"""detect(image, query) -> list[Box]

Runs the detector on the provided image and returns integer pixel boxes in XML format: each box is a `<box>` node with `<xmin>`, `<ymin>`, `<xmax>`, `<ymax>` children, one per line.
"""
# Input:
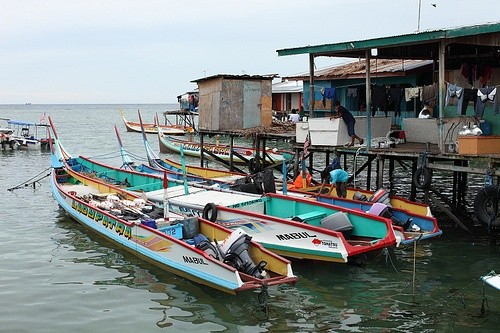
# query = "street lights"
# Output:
<box><xmin>416</xmin><ymin>0</ymin><xmax>437</xmax><ymax>32</ymax></box>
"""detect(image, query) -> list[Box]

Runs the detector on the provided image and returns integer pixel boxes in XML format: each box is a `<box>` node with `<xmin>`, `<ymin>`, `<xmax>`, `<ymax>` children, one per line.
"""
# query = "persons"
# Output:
<box><xmin>289</xmin><ymin>106</ymin><xmax>305</xmax><ymax>123</ymax></box>
<box><xmin>0</xmin><ymin>130</ymin><xmax>16</xmax><ymax>155</ymax></box>
<box><xmin>315</xmin><ymin>169</ymin><xmax>348</xmax><ymax>198</ymax></box>
<box><xmin>330</xmin><ymin>101</ymin><xmax>363</xmax><ymax>146</ymax></box>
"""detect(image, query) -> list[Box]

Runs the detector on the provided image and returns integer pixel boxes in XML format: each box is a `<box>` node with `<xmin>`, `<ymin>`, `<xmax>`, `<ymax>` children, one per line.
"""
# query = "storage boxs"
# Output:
<box><xmin>457</xmin><ymin>135</ymin><xmax>500</xmax><ymax>155</ymax></box>
<box><xmin>157</xmin><ymin>223</ymin><xmax>184</xmax><ymax>239</ymax></box>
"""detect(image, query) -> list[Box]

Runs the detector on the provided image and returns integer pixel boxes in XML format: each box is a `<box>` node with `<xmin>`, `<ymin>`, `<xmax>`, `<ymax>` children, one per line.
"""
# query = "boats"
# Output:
<box><xmin>0</xmin><ymin>117</ymin><xmax>55</xmax><ymax>151</ymax></box>
<box><xmin>118</xmin><ymin>111</ymin><xmax>194</xmax><ymax>135</ymax></box>
<box><xmin>155</xmin><ymin>111</ymin><xmax>297</xmax><ymax>166</ymax></box>
<box><xmin>48</xmin><ymin>108</ymin><xmax>444</xmax><ymax>296</ymax></box>
<box><xmin>480</xmin><ymin>270</ymin><xmax>500</xmax><ymax>291</ymax></box>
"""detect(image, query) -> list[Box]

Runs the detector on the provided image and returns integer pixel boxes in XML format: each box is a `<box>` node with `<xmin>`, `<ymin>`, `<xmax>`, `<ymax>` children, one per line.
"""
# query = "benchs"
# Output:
<box><xmin>288</xmin><ymin>211</ymin><xmax>326</xmax><ymax>226</ymax></box>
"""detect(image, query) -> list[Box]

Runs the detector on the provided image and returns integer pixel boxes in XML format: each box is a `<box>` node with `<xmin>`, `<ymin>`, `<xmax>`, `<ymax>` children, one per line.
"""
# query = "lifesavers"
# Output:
<box><xmin>202</xmin><ymin>203</ymin><xmax>217</xmax><ymax>223</ymax></box>
<box><xmin>413</xmin><ymin>168</ymin><xmax>431</xmax><ymax>188</ymax></box>
<box><xmin>248</xmin><ymin>158</ymin><xmax>259</xmax><ymax>175</ymax></box>
<box><xmin>474</xmin><ymin>185</ymin><xmax>500</xmax><ymax>230</ymax></box>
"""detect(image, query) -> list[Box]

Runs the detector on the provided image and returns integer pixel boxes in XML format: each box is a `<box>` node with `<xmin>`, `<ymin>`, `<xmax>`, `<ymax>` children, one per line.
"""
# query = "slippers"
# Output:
<box><xmin>360</xmin><ymin>139</ymin><xmax>363</xmax><ymax>145</ymax></box>
